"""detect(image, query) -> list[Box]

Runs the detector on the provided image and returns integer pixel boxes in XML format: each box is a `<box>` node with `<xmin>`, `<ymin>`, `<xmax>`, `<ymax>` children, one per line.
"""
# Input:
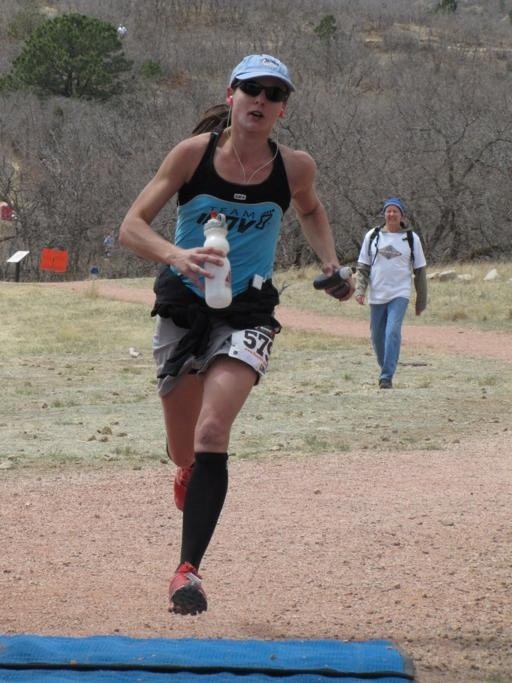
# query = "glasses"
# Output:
<box><xmin>234</xmin><ymin>80</ymin><xmax>289</xmax><ymax>102</ymax></box>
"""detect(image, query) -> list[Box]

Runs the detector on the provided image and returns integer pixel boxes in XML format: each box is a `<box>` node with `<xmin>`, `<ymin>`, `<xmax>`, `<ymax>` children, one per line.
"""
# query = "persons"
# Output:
<box><xmin>354</xmin><ymin>198</ymin><xmax>428</xmax><ymax>388</ymax></box>
<box><xmin>118</xmin><ymin>52</ymin><xmax>358</xmax><ymax>616</ymax></box>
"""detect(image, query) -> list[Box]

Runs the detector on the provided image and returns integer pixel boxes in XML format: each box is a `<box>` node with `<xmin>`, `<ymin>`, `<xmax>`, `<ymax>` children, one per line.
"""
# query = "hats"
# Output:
<box><xmin>229</xmin><ymin>54</ymin><xmax>297</xmax><ymax>93</ymax></box>
<box><xmin>382</xmin><ymin>198</ymin><xmax>405</xmax><ymax>217</ymax></box>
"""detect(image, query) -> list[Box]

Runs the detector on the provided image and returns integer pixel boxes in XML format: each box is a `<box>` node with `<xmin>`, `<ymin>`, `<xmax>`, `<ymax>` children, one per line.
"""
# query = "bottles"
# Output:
<box><xmin>199</xmin><ymin>208</ymin><xmax>233</xmax><ymax>314</ymax></box>
<box><xmin>311</xmin><ymin>266</ymin><xmax>357</xmax><ymax>290</ymax></box>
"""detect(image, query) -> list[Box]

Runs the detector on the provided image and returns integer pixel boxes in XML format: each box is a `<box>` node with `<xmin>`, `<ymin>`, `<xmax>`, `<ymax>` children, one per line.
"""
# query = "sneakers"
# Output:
<box><xmin>168</xmin><ymin>561</ymin><xmax>207</xmax><ymax>616</ymax></box>
<box><xmin>174</xmin><ymin>461</ymin><xmax>195</xmax><ymax>512</ymax></box>
<box><xmin>379</xmin><ymin>378</ymin><xmax>392</xmax><ymax>388</ymax></box>
